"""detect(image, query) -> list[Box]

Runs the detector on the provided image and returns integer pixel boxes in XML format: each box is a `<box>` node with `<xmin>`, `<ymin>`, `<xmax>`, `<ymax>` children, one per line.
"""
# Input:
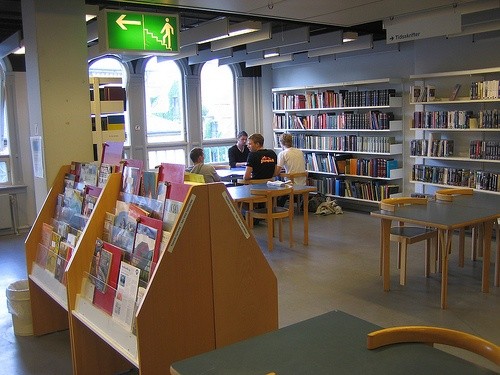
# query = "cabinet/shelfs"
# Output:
<box><xmin>410</xmin><ymin>67</ymin><xmax>500</xmax><ymax>194</ymax></box>
<box><xmin>272</xmin><ymin>78</ymin><xmax>405</xmax><ymax>204</ymax></box>
<box><xmin>88</xmin><ymin>76</ymin><xmax>126</xmax><ymax>162</ymax></box>
<box><xmin>24</xmin><ymin>164</ymin><xmax>277</xmax><ymax>375</ymax></box>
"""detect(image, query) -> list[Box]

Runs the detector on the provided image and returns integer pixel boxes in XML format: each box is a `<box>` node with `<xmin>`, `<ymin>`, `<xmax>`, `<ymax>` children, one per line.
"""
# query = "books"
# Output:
<box><xmin>409</xmin><ymin>80</ymin><xmax>500</xmax><ymax>191</ymax></box>
<box><xmin>35</xmin><ymin>86</ymin><xmax>192</xmax><ymax>334</ymax></box>
<box><xmin>271</xmin><ymin>88</ymin><xmax>399</xmax><ymax>201</ymax></box>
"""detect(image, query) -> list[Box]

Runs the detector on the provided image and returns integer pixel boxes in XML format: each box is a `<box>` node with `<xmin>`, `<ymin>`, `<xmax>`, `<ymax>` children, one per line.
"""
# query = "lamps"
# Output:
<box><xmin>343</xmin><ymin>32</ymin><xmax>358</xmax><ymax>43</ymax></box>
<box><xmin>196</xmin><ymin>20</ymin><xmax>262</xmax><ymax>45</ymax></box>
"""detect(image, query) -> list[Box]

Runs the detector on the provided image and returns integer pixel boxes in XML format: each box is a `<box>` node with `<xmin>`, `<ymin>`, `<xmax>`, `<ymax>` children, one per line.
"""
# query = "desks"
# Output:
<box><xmin>225</xmin><ymin>182</ymin><xmax>318</xmax><ymax>246</ymax></box>
<box><xmin>169</xmin><ymin>311</ymin><xmax>500</xmax><ymax>375</ymax></box>
<box><xmin>0</xmin><ymin>184</ymin><xmax>27</xmax><ymax>236</ymax></box>
<box><xmin>214</xmin><ymin>168</ymin><xmax>245</xmax><ymax>176</ymax></box>
<box><xmin>370</xmin><ymin>192</ymin><xmax>500</xmax><ymax>309</ymax></box>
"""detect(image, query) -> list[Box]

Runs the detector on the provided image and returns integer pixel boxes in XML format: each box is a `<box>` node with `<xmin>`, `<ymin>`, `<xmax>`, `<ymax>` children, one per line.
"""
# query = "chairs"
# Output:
<box><xmin>236</xmin><ymin>171</ymin><xmax>309</xmax><ymax>252</ymax></box>
<box><xmin>367</xmin><ymin>326</ymin><xmax>500</xmax><ymax>366</ymax></box>
<box><xmin>380</xmin><ymin>188</ymin><xmax>484</xmax><ymax>286</ymax></box>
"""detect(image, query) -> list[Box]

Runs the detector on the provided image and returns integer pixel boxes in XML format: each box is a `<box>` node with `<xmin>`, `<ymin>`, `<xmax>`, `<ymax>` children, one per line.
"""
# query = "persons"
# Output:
<box><xmin>228</xmin><ymin>130</ymin><xmax>250</xmax><ymax>182</ymax></box>
<box><xmin>190</xmin><ymin>147</ymin><xmax>220</xmax><ymax>183</ymax></box>
<box><xmin>242</xmin><ymin>133</ymin><xmax>277</xmax><ymax>224</ymax></box>
<box><xmin>277</xmin><ymin>133</ymin><xmax>306</xmax><ymax>206</ymax></box>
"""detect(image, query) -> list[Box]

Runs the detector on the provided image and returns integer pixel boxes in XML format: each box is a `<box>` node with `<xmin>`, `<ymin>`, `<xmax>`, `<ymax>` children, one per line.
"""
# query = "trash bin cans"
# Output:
<box><xmin>6</xmin><ymin>279</ymin><xmax>34</xmax><ymax>337</ymax></box>
